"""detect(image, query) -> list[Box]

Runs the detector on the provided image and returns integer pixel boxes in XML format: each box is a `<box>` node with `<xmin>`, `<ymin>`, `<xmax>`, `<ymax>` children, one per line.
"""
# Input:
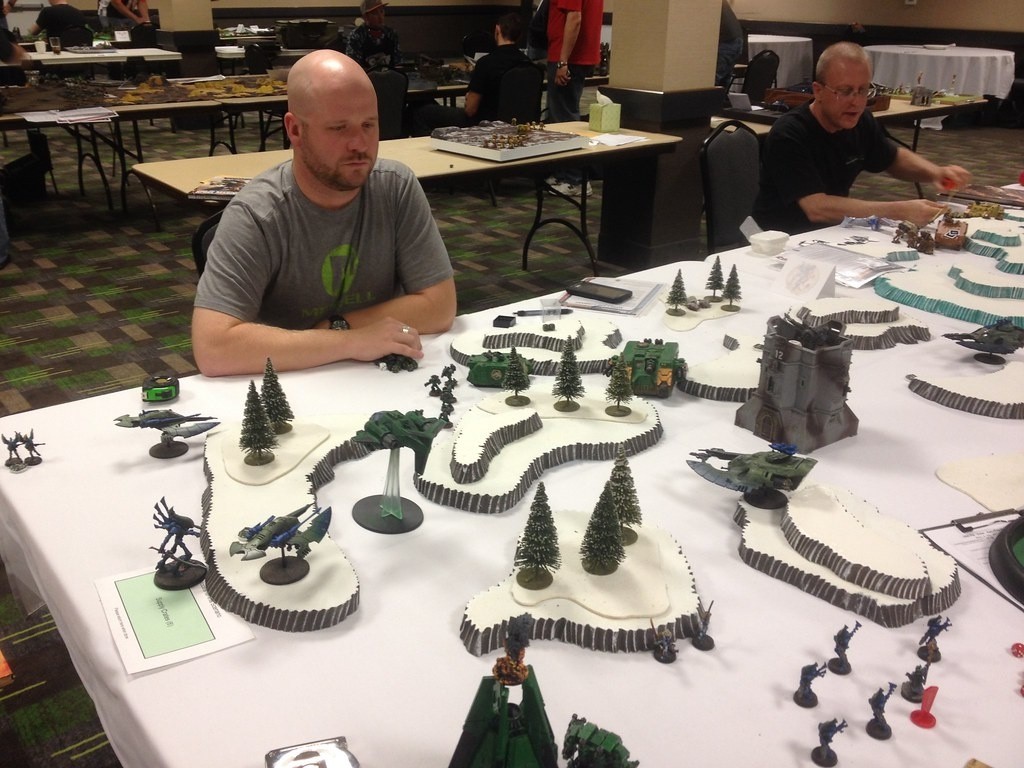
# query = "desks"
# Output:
<box><xmin>866</xmin><ymin>44</ymin><xmax>1017</xmax><ymax>132</ymax></box>
<box><xmin>132</xmin><ymin>119</ymin><xmax>686</xmax><ymax>280</ymax></box>
<box><xmin>0</xmin><ymin>85</ymin><xmax>223</xmax><ymax>224</ymax></box>
<box><xmin>710</xmin><ymin>97</ymin><xmax>989</xmax><ymax>221</ymax></box>
<box><xmin>747</xmin><ymin>32</ymin><xmax>813</xmax><ymax>90</ymax></box>
<box><xmin>215</xmin><ymin>43</ymin><xmax>318</xmax><ymax>57</ymax></box>
<box><xmin>205</xmin><ymin>72</ymin><xmax>292</xmax><ymax>156</ymax></box>
<box><xmin>217</xmin><ymin>35</ymin><xmax>276</xmax><ymax>42</ymax></box>
<box><xmin>38</xmin><ymin>46</ymin><xmax>184</xmax><ymax>80</ymax></box>
<box><xmin>0</xmin><ymin>184</ymin><xmax>1024</xmax><ymax>768</ymax></box>
<box><xmin>404</xmin><ymin>76</ymin><xmax>609</xmax><ymax>107</ymax></box>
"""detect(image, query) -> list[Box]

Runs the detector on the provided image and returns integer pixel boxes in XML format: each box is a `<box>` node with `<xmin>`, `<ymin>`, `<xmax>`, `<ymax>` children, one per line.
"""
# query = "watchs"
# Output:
<box><xmin>328</xmin><ymin>314</ymin><xmax>350</xmax><ymax>330</ymax></box>
<box><xmin>557</xmin><ymin>60</ymin><xmax>567</xmax><ymax>68</ymax></box>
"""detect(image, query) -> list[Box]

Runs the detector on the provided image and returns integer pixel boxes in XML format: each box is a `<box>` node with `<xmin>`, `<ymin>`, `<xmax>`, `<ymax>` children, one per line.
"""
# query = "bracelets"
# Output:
<box><xmin>8</xmin><ymin>1</ymin><xmax>14</xmax><ymax>8</ymax></box>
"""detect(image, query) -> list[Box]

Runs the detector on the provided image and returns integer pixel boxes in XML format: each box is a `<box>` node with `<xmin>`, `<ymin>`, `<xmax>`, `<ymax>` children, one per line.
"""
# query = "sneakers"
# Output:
<box><xmin>542</xmin><ymin>176</ymin><xmax>569</xmax><ymax>193</ymax></box>
<box><xmin>549</xmin><ymin>181</ymin><xmax>593</xmax><ymax>196</ymax></box>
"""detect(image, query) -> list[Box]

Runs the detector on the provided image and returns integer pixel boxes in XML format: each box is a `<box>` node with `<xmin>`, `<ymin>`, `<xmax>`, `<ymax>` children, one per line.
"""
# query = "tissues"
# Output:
<box><xmin>588</xmin><ymin>89</ymin><xmax>621</xmax><ymax>133</ymax></box>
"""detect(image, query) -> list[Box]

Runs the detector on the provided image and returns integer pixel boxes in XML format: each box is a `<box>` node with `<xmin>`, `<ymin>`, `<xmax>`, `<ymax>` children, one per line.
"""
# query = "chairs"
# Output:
<box><xmin>700</xmin><ymin>121</ymin><xmax>766</xmax><ymax>249</ymax></box>
<box><xmin>446</xmin><ymin>58</ymin><xmax>542</xmax><ymax>206</ymax></box>
<box><xmin>59</xmin><ymin>26</ymin><xmax>94</xmax><ymax>49</ymax></box>
<box><xmin>130</xmin><ymin>22</ymin><xmax>160</xmax><ymax>46</ymax></box>
<box><xmin>243</xmin><ymin>42</ymin><xmax>273</xmax><ymax>75</ymax></box>
<box><xmin>191</xmin><ymin>201</ymin><xmax>230</xmax><ymax>278</ymax></box>
<box><xmin>368</xmin><ymin>64</ymin><xmax>409</xmax><ymax>137</ymax></box>
<box><xmin>734</xmin><ymin>47</ymin><xmax>782</xmax><ymax>99</ymax></box>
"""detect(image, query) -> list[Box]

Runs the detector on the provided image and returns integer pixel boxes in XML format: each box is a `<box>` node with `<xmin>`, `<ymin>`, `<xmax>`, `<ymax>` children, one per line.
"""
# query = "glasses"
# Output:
<box><xmin>818</xmin><ymin>80</ymin><xmax>877</xmax><ymax>105</ymax></box>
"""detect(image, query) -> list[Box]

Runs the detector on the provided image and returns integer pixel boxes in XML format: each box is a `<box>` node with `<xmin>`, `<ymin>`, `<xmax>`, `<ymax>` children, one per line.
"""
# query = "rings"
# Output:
<box><xmin>402</xmin><ymin>325</ymin><xmax>409</xmax><ymax>334</ymax></box>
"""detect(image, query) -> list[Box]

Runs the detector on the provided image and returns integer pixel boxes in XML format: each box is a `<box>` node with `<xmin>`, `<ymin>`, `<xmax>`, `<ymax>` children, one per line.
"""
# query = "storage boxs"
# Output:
<box><xmin>274</xmin><ymin>17</ymin><xmax>340</xmax><ymax>47</ymax></box>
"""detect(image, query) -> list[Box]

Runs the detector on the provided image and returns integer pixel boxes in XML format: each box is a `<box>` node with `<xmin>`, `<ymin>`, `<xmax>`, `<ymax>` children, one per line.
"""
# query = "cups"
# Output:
<box><xmin>49</xmin><ymin>36</ymin><xmax>61</xmax><ymax>55</ymax></box>
<box><xmin>34</xmin><ymin>41</ymin><xmax>46</xmax><ymax>54</ymax></box>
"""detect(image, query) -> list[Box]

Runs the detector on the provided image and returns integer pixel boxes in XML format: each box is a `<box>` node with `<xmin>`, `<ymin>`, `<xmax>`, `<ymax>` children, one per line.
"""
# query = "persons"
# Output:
<box><xmin>97</xmin><ymin>0</ymin><xmax>150</xmax><ymax>29</ymax></box>
<box><xmin>413</xmin><ymin>12</ymin><xmax>543</xmax><ymax>137</ymax></box>
<box><xmin>192</xmin><ymin>49</ymin><xmax>456</xmax><ymax>377</ymax></box>
<box><xmin>751</xmin><ymin>41</ymin><xmax>971</xmax><ymax>228</ymax></box>
<box><xmin>31</xmin><ymin>0</ymin><xmax>86</xmax><ymax>51</ymax></box>
<box><xmin>345</xmin><ymin>0</ymin><xmax>402</xmax><ymax>73</ymax></box>
<box><xmin>538</xmin><ymin>0</ymin><xmax>604</xmax><ymax>123</ymax></box>
<box><xmin>0</xmin><ymin>0</ymin><xmax>26</xmax><ymax>64</ymax></box>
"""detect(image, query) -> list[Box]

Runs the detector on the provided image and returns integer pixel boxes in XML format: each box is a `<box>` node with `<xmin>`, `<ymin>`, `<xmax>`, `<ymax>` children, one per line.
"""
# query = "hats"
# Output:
<box><xmin>361</xmin><ymin>0</ymin><xmax>389</xmax><ymax>15</ymax></box>
